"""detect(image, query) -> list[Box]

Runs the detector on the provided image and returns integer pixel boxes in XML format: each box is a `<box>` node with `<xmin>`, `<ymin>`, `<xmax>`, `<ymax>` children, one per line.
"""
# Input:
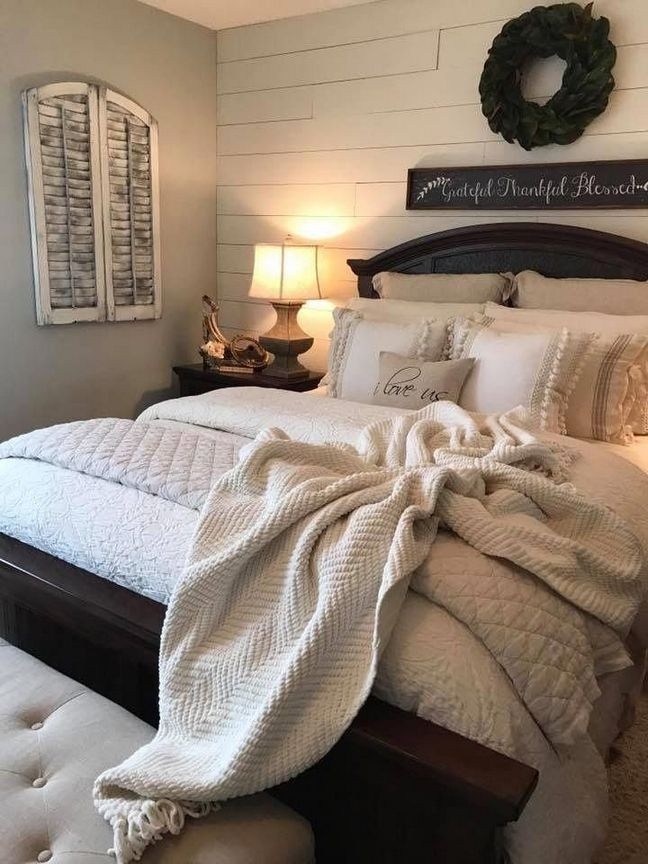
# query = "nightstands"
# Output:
<box><xmin>172</xmin><ymin>364</ymin><xmax>326</xmax><ymax>398</ymax></box>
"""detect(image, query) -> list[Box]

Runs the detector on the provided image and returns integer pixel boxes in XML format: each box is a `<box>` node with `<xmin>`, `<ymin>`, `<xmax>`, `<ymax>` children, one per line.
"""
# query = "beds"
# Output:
<box><xmin>0</xmin><ymin>222</ymin><xmax>648</xmax><ymax>864</ymax></box>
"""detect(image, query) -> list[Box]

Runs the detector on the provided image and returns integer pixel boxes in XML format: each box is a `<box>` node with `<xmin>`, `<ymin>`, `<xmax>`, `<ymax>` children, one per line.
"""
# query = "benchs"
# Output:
<box><xmin>0</xmin><ymin>637</ymin><xmax>317</xmax><ymax>864</ymax></box>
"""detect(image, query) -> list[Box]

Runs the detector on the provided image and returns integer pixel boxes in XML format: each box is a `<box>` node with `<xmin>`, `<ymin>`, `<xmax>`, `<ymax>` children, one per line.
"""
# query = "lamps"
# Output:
<box><xmin>247</xmin><ymin>234</ymin><xmax>329</xmax><ymax>378</ymax></box>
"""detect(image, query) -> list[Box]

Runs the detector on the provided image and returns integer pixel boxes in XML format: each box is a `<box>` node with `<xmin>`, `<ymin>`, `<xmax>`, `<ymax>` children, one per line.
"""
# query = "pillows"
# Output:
<box><xmin>318</xmin><ymin>270</ymin><xmax>647</xmax><ymax>445</ymax></box>
